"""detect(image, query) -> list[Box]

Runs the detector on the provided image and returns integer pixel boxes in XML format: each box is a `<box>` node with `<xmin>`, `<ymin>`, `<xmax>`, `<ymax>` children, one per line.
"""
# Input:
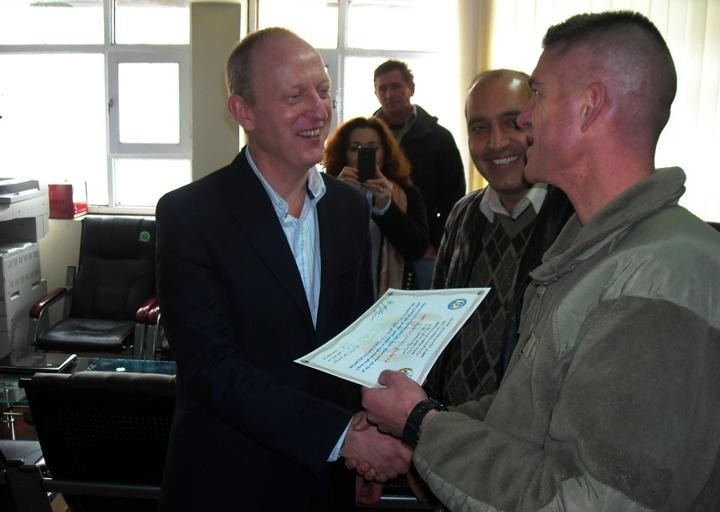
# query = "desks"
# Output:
<box><xmin>2</xmin><ymin>347</ymin><xmax>178</xmax><ymax>442</ymax></box>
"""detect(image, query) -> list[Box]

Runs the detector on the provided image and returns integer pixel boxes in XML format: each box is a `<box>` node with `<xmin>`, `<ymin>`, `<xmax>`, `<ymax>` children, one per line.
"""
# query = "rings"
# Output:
<box><xmin>378</xmin><ymin>185</ymin><xmax>385</xmax><ymax>194</ymax></box>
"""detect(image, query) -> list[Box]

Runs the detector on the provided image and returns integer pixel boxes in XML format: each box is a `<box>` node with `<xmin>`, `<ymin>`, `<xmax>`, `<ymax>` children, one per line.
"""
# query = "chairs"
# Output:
<box><xmin>1</xmin><ymin>370</ymin><xmax>177</xmax><ymax>511</ymax></box>
<box><xmin>29</xmin><ymin>217</ymin><xmax>171</xmax><ymax>361</ymax></box>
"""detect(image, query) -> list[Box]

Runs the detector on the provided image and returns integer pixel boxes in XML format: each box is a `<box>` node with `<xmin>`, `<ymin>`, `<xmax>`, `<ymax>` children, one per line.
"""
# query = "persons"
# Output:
<box><xmin>367</xmin><ymin>62</ymin><xmax>458</xmax><ymax>261</ymax></box>
<box><xmin>365</xmin><ymin>11</ymin><xmax>720</xmax><ymax>512</ymax></box>
<box><xmin>403</xmin><ymin>68</ymin><xmax>572</xmax><ymax>512</ymax></box>
<box><xmin>320</xmin><ymin>115</ymin><xmax>430</xmax><ymax>302</ymax></box>
<box><xmin>155</xmin><ymin>28</ymin><xmax>414</xmax><ymax>511</ymax></box>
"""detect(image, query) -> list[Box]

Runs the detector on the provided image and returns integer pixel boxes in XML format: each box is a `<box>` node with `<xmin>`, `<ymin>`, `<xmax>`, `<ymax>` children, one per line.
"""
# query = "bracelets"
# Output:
<box><xmin>401</xmin><ymin>396</ymin><xmax>450</xmax><ymax>451</ymax></box>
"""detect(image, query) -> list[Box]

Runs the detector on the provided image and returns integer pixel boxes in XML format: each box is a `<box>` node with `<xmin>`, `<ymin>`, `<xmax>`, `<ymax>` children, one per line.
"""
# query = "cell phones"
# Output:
<box><xmin>356</xmin><ymin>147</ymin><xmax>376</xmax><ymax>183</ymax></box>
